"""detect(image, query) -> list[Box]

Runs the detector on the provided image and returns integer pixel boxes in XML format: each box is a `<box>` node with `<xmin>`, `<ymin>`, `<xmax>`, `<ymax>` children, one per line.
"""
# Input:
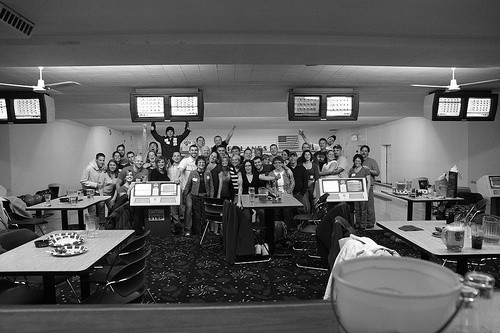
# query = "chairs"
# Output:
<box><xmin>440</xmin><ymin>198</ymin><xmax>499</xmax><ymax>274</ymax></box>
<box><xmin>199</xmin><ymin>196</ymin><xmax>275</xmax><ymax>264</ymax></box>
<box><xmin>289</xmin><ymin>192</ymin><xmax>355</xmax><ymax>274</ymax></box>
<box><xmin>0</xmin><ymin>196</ymin><xmax>157</xmax><ymax>305</ymax></box>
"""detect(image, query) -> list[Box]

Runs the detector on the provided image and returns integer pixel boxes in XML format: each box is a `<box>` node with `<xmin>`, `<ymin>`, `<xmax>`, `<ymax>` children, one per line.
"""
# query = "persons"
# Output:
<box><xmin>78</xmin><ymin>130</ymin><xmax>380</xmax><ymax>236</ymax></box>
<box><xmin>212</xmin><ymin>123</ymin><xmax>236</xmax><ymax>152</ymax></box>
<box><xmin>150</xmin><ymin>122</ymin><xmax>192</xmax><ymax>164</ymax></box>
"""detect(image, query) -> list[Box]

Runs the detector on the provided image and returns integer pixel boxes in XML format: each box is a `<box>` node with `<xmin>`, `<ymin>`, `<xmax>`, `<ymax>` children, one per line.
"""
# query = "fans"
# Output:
<box><xmin>409</xmin><ymin>68</ymin><xmax>500</xmax><ymax>93</ymax></box>
<box><xmin>0</xmin><ymin>65</ymin><xmax>82</xmax><ymax>96</ymax></box>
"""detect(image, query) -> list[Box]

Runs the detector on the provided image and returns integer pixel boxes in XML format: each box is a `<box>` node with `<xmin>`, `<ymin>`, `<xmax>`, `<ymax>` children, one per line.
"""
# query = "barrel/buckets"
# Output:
<box><xmin>330</xmin><ymin>256</ymin><xmax>465</xmax><ymax>333</ymax></box>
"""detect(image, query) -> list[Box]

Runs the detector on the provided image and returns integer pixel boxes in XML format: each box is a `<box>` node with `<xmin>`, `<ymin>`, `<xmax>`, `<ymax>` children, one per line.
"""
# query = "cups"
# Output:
<box><xmin>85</xmin><ymin>213</ymin><xmax>100</xmax><ymax>238</ymax></box>
<box><xmin>440</xmin><ymin>223</ymin><xmax>465</xmax><ymax>252</ymax></box>
<box><xmin>249</xmin><ymin>187</ymin><xmax>282</xmax><ymax>203</ymax></box>
<box><xmin>392</xmin><ymin>178</ymin><xmax>447</xmax><ymax>198</ymax></box>
<box><xmin>44</xmin><ymin>187</ymin><xmax>94</xmax><ymax>206</ymax></box>
<box><xmin>299</xmin><ymin>129</ymin><xmax>304</xmax><ymax>135</ymax></box>
<box><xmin>471</xmin><ymin>223</ymin><xmax>486</xmax><ymax>249</ymax></box>
<box><xmin>446</xmin><ymin>208</ymin><xmax>455</xmax><ymax>224</ymax></box>
<box><xmin>453</xmin><ymin>271</ymin><xmax>496</xmax><ymax>333</ymax></box>
<box><xmin>481</xmin><ymin>215</ymin><xmax>500</xmax><ymax>244</ymax></box>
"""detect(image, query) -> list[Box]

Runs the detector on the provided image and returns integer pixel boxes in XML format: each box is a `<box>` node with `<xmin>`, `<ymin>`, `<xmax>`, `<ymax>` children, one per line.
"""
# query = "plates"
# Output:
<box><xmin>46</xmin><ymin>232</ymin><xmax>88</xmax><ymax>258</ymax></box>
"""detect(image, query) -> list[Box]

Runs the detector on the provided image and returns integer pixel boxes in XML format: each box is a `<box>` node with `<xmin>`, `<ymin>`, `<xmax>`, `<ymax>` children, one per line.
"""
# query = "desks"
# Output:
<box><xmin>241</xmin><ymin>192</ymin><xmax>304</xmax><ymax>256</ymax></box>
<box><xmin>375</xmin><ymin>220</ymin><xmax>500</xmax><ymax>277</ymax></box>
<box><xmin>25</xmin><ymin>195</ymin><xmax>112</xmax><ymax>230</ymax></box>
<box><xmin>0</xmin><ymin>230</ymin><xmax>135</xmax><ymax>304</ymax></box>
<box><xmin>381</xmin><ymin>189</ymin><xmax>464</xmax><ymax>221</ymax></box>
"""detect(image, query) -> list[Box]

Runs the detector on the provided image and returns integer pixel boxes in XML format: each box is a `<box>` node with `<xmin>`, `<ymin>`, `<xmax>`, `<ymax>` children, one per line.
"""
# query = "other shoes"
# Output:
<box><xmin>184</xmin><ymin>230</ymin><xmax>191</xmax><ymax>236</ymax></box>
<box><xmin>354</xmin><ymin>225</ymin><xmax>357</xmax><ymax>230</ymax></box>
<box><xmin>358</xmin><ymin>227</ymin><xmax>364</xmax><ymax>231</ymax></box>
<box><xmin>366</xmin><ymin>225</ymin><xmax>374</xmax><ymax>228</ymax></box>
